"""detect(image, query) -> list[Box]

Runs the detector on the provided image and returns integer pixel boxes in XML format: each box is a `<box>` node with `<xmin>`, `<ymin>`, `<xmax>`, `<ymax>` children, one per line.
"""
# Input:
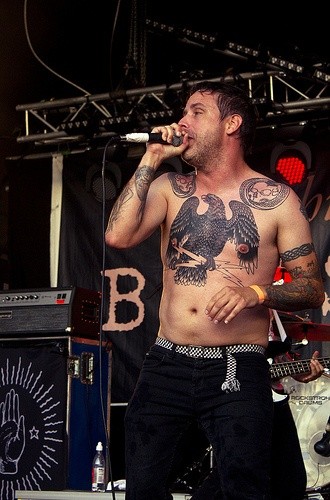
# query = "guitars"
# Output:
<box><xmin>267</xmin><ymin>351</ymin><xmax>330</xmax><ymax>402</ymax></box>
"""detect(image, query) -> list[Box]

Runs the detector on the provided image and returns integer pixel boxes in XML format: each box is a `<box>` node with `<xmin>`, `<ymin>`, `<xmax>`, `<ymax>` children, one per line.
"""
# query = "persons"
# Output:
<box><xmin>266</xmin><ymin>307</ymin><xmax>324</xmax><ymax>500</ymax></box>
<box><xmin>314</xmin><ymin>416</ymin><xmax>330</xmax><ymax>457</ymax></box>
<box><xmin>104</xmin><ymin>79</ymin><xmax>326</xmax><ymax>500</ymax></box>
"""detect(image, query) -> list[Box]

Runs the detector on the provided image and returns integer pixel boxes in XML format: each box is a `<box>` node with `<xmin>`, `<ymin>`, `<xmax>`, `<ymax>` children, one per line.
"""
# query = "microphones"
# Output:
<box><xmin>114</xmin><ymin>132</ymin><xmax>184</xmax><ymax>146</ymax></box>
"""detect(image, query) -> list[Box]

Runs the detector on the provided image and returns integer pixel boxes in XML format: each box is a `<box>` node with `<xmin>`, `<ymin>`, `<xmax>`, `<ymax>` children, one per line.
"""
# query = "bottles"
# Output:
<box><xmin>91</xmin><ymin>441</ymin><xmax>106</xmax><ymax>492</ymax></box>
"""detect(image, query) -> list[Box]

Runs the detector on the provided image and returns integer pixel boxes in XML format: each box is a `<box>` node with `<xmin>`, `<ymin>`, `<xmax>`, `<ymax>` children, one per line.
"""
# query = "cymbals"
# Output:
<box><xmin>281</xmin><ymin>319</ymin><xmax>330</xmax><ymax>343</ymax></box>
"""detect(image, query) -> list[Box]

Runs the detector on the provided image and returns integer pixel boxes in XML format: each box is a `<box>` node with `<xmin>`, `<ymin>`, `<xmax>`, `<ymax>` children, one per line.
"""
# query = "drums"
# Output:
<box><xmin>266</xmin><ymin>310</ymin><xmax>290</xmax><ymax>359</ymax></box>
<box><xmin>280</xmin><ymin>367</ymin><xmax>330</xmax><ymax>492</ymax></box>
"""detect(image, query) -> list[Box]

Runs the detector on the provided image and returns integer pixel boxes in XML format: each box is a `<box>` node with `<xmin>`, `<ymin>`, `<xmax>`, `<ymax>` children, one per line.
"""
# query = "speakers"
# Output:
<box><xmin>0</xmin><ymin>338</ymin><xmax>111</xmax><ymax>500</ymax></box>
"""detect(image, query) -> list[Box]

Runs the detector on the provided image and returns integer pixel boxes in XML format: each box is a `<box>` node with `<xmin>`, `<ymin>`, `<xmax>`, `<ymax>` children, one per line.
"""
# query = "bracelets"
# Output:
<box><xmin>249</xmin><ymin>284</ymin><xmax>264</xmax><ymax>305</ymax></box>
